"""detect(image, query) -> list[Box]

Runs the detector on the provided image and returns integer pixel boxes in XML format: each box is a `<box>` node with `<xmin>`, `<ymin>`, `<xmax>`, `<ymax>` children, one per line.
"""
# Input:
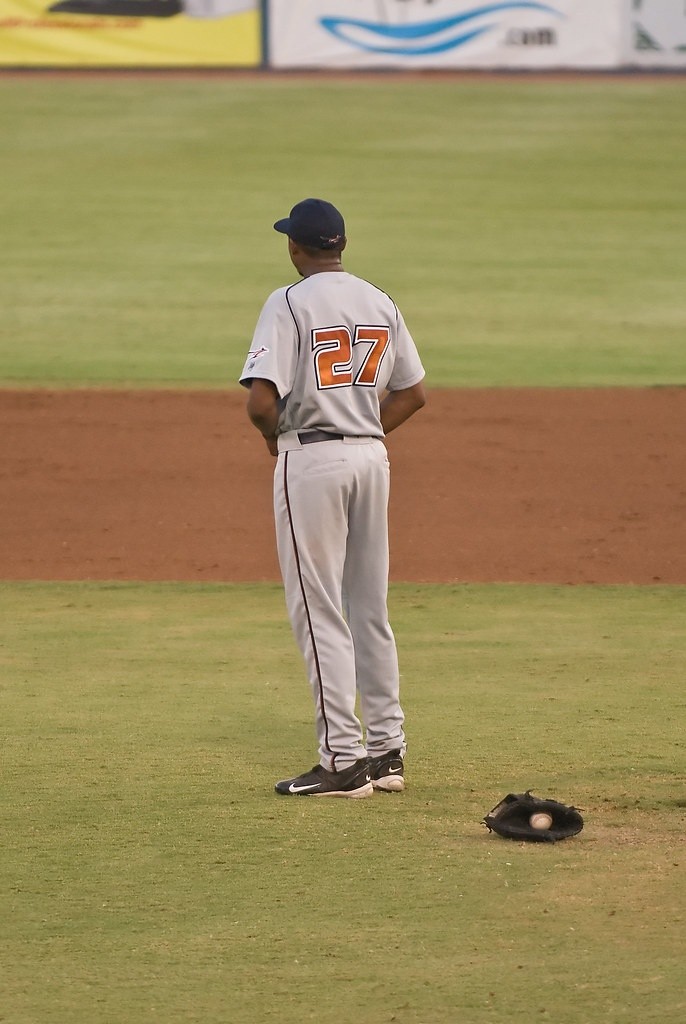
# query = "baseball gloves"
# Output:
<box><xmin>482</xmin><ymin>791</ymin><xmax>583</xmax><ymax>842</ymax></box>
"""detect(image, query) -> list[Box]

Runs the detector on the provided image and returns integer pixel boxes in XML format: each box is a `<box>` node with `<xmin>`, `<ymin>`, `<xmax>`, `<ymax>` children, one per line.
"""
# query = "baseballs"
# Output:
<box><xmin>526</xmin><ymin>812</ymin><xmax>553</xmax><ymax>831</ymax></box>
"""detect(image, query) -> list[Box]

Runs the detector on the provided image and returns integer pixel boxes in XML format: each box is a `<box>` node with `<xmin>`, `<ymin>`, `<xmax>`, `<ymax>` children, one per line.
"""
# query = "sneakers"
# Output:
<box><xmin>368</xmin><ymin>748</ymin><xmax>405</xmax><ymax>792</ymax></box>
<box><xmin>275</xmin><ymin>757</ymin><xmax>373</xmax><ymax>799</ymax></box>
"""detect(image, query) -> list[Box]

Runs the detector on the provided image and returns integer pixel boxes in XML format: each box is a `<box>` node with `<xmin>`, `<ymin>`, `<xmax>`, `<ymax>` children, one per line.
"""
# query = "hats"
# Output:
<box><xmin>273</xmin><ymin>198</ymin><xmax>346</xmax><ymax>250</ymax></box>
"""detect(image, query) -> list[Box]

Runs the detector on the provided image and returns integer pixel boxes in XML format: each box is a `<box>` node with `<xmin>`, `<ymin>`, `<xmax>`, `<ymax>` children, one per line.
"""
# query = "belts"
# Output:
<box><xmin>297</xmin><ymin>428</ymin><xmax>380</xmax><ymax>442</ymax></box>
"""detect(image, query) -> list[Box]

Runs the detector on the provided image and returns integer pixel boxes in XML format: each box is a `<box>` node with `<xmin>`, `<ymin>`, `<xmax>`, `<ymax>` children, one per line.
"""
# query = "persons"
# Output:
<box><xmin>239</xmin><ymin>198</ymin><xmax>427</xmax><ymax>799</ymax></box>
